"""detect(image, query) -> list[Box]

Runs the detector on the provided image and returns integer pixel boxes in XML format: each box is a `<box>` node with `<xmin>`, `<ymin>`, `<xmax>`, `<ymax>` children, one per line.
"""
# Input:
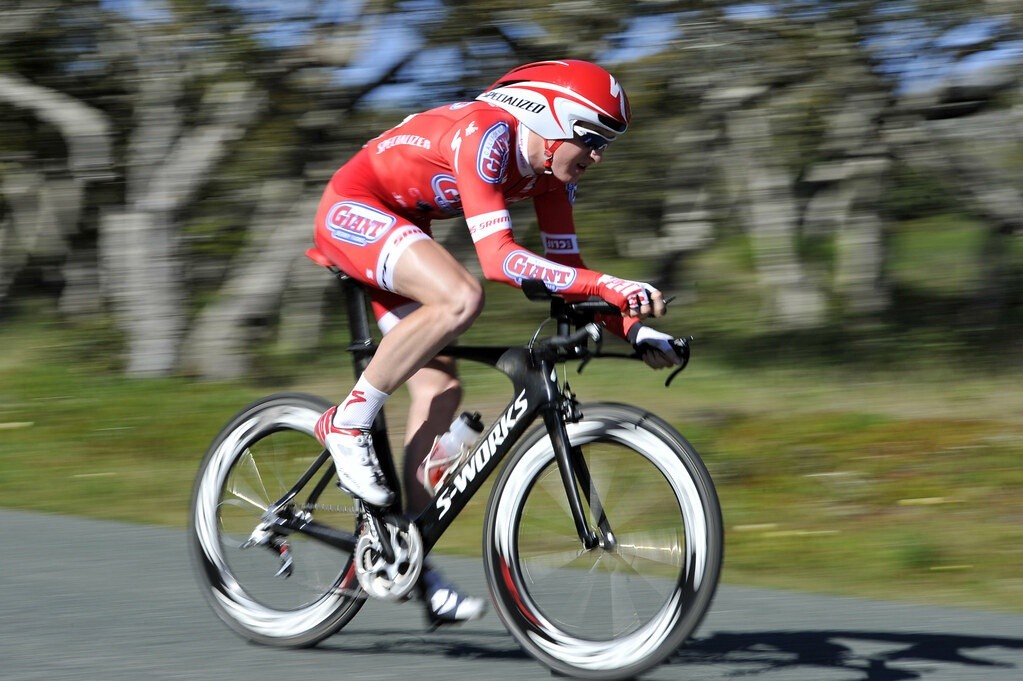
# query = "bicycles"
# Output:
<box><xmin>186</xmin><ymin>246</ymin><xmax>725</xmax><ymax>681</ymax></box>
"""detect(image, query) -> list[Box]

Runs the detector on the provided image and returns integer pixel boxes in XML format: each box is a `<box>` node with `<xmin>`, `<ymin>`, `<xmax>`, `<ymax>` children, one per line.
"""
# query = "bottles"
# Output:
<box><xmin>418</xmin><ymin>412</ymin><xmax>485</xmax><ymax>492</ymax></box>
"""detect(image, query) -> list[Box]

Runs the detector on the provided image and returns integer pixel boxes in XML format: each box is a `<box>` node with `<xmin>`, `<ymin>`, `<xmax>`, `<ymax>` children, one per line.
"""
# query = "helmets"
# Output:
<box><xmin>474</xmin><ymin>59</ymin><xmax>632</xmax><ymax>139</ymax></box>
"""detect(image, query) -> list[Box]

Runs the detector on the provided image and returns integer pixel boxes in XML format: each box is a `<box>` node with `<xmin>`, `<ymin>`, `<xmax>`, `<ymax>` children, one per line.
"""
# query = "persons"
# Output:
<box><xmin>312</xmin><ymin>59</ymin><xmax>682</xmax><ymax>622</ymax></box>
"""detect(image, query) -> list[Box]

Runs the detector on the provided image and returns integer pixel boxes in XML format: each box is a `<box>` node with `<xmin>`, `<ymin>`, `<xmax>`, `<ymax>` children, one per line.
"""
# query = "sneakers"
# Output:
<box><xmin>314</xmin><ymin>406</ymin><xmax>395</xmax><ymax>507</ymax></box>
<box><xmin>425</xmin><ymin>582</ymin><xmax>484</xmax><ymax>623</ymax></box>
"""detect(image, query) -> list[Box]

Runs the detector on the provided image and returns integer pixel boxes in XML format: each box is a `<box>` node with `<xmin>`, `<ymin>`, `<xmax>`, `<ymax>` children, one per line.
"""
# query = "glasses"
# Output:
<box><xmin>574</xmin><ymin>125</ymin><xmax>618</xmax><ymax>151</ymax></box>
